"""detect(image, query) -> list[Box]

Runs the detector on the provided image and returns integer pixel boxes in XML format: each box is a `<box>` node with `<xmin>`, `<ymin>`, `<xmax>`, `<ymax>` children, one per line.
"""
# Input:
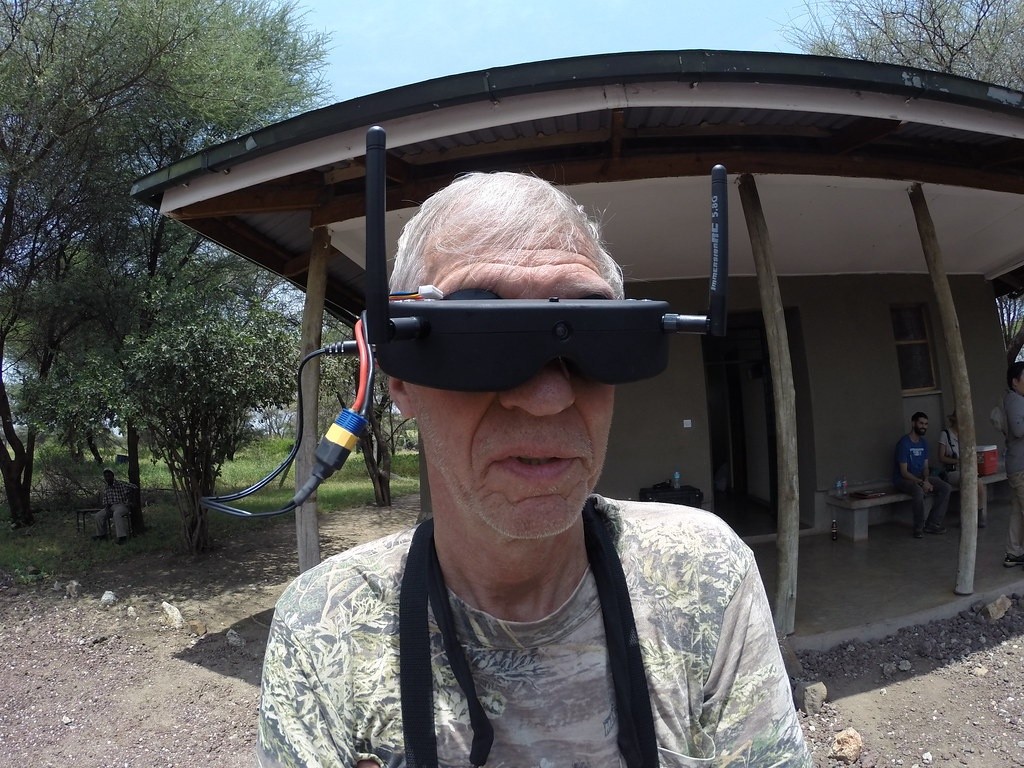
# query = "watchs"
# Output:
<box><xmin>919</xmin><ymin>481</ymin><xmax>925</xmax><ymax>487</ymax></box>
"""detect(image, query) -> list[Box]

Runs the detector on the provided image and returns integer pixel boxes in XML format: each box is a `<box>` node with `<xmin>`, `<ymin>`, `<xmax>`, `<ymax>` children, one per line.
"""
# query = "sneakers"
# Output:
<box><xmin>1003</xmin><ymin>551</ymin><xmax>1024</xmax><ymax>567</ymax></box>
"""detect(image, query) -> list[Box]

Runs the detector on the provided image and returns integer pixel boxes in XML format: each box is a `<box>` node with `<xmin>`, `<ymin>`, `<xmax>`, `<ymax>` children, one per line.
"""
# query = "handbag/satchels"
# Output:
<box><xmin>942</xmin><ymin>429</ymin><xmax>957</xmax><ymax>471</ymax></box>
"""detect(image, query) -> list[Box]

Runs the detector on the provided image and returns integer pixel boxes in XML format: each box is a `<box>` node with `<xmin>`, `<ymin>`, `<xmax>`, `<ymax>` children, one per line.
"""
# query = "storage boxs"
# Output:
<box><xmin>976</xmin><ymin>444</ymin><xmax>999</xmax><ymax>474</ymax></box>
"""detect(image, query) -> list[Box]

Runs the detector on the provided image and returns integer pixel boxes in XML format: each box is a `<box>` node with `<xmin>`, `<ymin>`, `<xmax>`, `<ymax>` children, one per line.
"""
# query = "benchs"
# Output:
<box><xmin>828</xmin><ymin>465</ymin><xmax>1007</xmax><ymax>540</ymax></box>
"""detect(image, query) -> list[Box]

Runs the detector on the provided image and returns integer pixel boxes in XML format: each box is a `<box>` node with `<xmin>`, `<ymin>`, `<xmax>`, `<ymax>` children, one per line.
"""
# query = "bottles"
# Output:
<box><xmin>835</xmin><ymin>480</ymin><xmax>842</xmax><ymax>497</ymax></box>
<box><xmin>674</xmin><ymin>471</ymin><xmax>680</xmax><ymax>488</ymax></box>
<box><xmin>842</xmin><ymin>480</ymin><xmax>848</xmax><ymax>495</ymax></box>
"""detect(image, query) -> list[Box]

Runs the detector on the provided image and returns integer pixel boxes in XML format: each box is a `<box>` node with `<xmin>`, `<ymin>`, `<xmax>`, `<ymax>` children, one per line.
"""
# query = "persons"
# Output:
<box><xmin>1001</xmin><ymin>360</ymin><xmax>1024</xmax><ymax>567</ymax></box>
<box><xmin>258</xmin><ymin>169</ymin><xmax>817</xmax><ymax>768</ymax></box>
<box><xmin>938</xmin><ymin>410</ymin><xmax>986</xmax><ymax>527</ymax></box>
<box><xmin>89</xmin><ymin>466</ymin><xmax>138</xmax><ymax>545</ymax></box>
<box><xmin>891</xmin><ymin>411</ymin><xmax>952</xmax><ymax>537</ymax></box>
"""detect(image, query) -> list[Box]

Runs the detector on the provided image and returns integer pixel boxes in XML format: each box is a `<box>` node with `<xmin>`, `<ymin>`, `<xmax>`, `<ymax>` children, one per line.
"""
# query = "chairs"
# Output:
<box><xmin>77</xmin><ymin>492</ymin><xmax>136</xmax><ymax>541</ymax></box>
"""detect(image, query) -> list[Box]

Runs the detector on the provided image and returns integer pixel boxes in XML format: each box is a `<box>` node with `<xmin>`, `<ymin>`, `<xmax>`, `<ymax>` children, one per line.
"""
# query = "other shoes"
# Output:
<box><xmin>116</xmin><ymin>537</ymin><xmax>127</xmax><ymax>545</ymax></box>
<box><xmin>914</xmin><ymin>526</ymin><xmax>924</xmax><ymax>538</ymax></box>
<box><xmin>924</xmin><ymin>524</ymin><xmax>946</xmax><ymax>534</ymax></box>
<box><xmin>91</xmin><ymin>535</ymin><xmax>108</xmax><ymax>541</ymax></box>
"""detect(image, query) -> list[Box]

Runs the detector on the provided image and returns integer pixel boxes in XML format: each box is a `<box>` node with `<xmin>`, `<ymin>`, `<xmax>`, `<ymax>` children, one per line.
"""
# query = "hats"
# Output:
<box><xmin>989</xmin><ymin>396</ymin><xmax>1009</xmax><ymax>439</ymax></box>
